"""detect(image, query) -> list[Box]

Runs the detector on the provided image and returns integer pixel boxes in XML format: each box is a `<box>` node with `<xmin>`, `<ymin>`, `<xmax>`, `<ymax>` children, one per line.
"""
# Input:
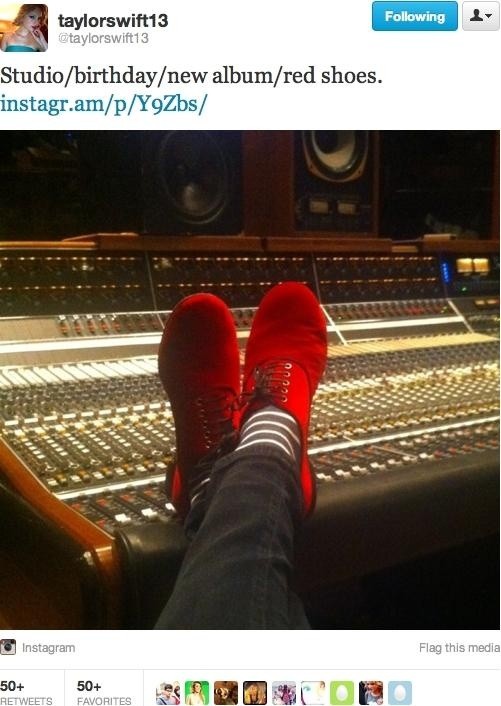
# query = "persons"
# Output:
<box><xmin>153</xmin><ymin>281</ymin><xmax>328</xmax><ymax>629</ymax></box>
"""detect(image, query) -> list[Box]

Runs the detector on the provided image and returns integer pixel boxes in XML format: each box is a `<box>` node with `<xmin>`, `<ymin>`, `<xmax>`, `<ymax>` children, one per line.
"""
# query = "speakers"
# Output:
<box><xmin>138</xmin><ymin>130</ymin><xmax>244</xmax><ymax>235</ymax></box>
<box><xmin>287</xmin><ymin>131</ymin><xmax>394</xmax><ymax>238</ymax></box>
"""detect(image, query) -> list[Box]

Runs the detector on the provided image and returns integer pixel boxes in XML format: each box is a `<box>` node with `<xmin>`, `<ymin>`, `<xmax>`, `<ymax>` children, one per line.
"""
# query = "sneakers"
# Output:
<box><xmin>236</xmin><ymin>281</ymin><xmax>329</xmax><ymax>526</ymax></box>
<box><xmin>156</xmin><ymin>292</ymin><xmax>243</xmax><ymax>517</ymax></box>
